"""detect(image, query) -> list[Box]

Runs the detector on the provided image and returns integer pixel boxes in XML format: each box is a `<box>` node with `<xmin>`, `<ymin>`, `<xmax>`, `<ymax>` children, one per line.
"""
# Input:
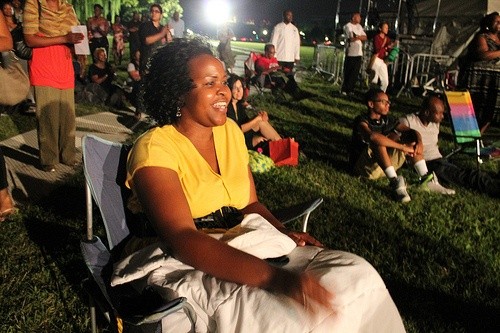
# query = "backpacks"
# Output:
<box><xmin>247</xmin><ymin>150</ymin><xmax>275</xmax><ymax>173</ymax></box>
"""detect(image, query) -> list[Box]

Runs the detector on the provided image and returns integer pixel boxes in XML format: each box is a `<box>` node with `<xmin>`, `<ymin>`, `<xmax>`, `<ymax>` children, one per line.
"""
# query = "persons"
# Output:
<box><xmin>368</xmin><ymin>22</ymin><xmax>397</xmax><ymax>93</ymax></box>
<box><xmin>255</xmin><ymin>44</ymin><xmax>308</xmax><ymax>102</ymax></box>
<box><xmin>0</xmin><ymin>0</ymin><xmax>185</xmax><ymax>222</ymax></box>
<box><xmin>397</xmin><ymin>95</ymin><xmax>500</xmax><ymax>199</ymax></box>
<box><xmin>227</xmin><ymin>75</ymin><xmax>286</xmax><ymax>153</ymax></box>
<box><xmin>120</xmin><ymin>37</ymin><xmax>406</xmax><ymax>333</ymax></box>
<box><xmin>218</xmin><ymin>23</ymin><xmax>235</xmax><ymax>73</ymax></box>
<box><xmin>350</xmin><ymin>88</ymin><xmax>454</xmax><ymax>205</ymax></box>
<box><xmin>270</xmin><ymin>9</ymin><xmax>300</xmax><ymax>72</ymax></box>
<box><xmin>474</xmin><ymin>11</ymin><xmax>500</xmax><ymax>124</ymax></box>
<box><xmin>342</xmin><ymin>12</ymin><xmax>367</xmax><ymax>96</ymax></box>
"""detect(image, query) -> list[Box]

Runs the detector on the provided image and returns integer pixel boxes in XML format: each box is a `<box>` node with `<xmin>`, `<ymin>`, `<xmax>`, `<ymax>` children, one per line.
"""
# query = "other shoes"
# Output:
<box><xmin>59</xmin><ymin>161</ymin><xmax>79</xmax><ymax>166</ymax></box>
<box><xmin>342</xmin><ymin>92</ymin><xmax>355</xmax><ymax>97</ymax></box>
<box><xmin>39</xmin><ymin>163</ymin><xmax>55</xmax><ymax>172</ymax></box>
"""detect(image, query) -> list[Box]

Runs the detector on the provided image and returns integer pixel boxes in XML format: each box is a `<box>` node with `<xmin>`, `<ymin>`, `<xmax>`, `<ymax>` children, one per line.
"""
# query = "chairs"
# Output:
<box><xmin>244</xmin><ymin>52</ymin><xmax>289</xmax><ymax>103</ymax></box>
<box><xmin>440</xmin><ymin>89</ymin><xmax>500</xmax><ymax>190</ymax></box>
<box><xmin>74</xmin><ymin>135</ymin><xmax>323</xmax><ymax>333</ymax></box>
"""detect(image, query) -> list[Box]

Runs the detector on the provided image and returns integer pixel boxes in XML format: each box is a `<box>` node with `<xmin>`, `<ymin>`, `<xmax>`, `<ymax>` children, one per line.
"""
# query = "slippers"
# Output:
<box><xmin>0</xmin><ymin>208</ymin><xmax>14</xmax><ymax>221</ymax></box>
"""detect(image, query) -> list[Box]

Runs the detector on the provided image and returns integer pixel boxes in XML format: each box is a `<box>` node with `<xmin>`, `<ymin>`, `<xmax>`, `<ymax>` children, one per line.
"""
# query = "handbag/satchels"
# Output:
<box><xmin>259</xmin><ymin>134</ymin><xmax>299</xmax><ymax>167</ymax></box>
<box><xmin>365</xmin><ymin>54</ymin><xmax>378</xmax><ymax>74</ymax></box>
<box><xmin>0</xmin><ymin>57</ymin><xmax>30</xmax><ymax>105</ymax></box>
<box><xmin>10</xmin><ymin>0</ymin><xmax>41</xmax><ymax>60</ymax></box>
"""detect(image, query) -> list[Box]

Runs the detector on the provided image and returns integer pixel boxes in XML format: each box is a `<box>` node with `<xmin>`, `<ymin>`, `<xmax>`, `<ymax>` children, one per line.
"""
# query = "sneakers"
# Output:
<box><xmin>392</xmin><ymin>176</ymin><xmax>412</xmax><ymax>203</ymax></box>
<box><xmin>418</xmin><ymin>173</ymin><xmax>455</xmax><ymax>196</ymax></box>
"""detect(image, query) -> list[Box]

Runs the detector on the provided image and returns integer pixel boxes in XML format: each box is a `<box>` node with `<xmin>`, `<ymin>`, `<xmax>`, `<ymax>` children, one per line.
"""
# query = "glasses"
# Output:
<box><xmin>150</xmin><ymin>9</ymin><xmax>160</xmax><ymax>13</ymax></box>
